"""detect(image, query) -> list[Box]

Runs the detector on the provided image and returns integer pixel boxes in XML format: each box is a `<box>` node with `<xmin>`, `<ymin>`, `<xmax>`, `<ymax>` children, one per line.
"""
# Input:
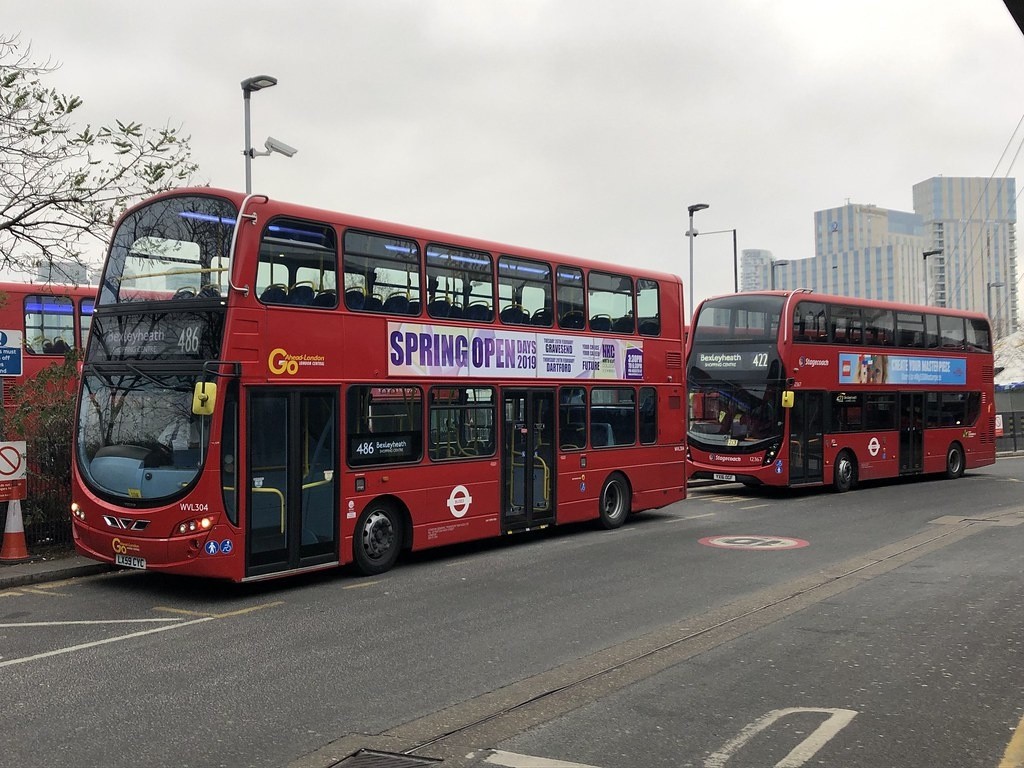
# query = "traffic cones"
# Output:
<box><xmin>0</xmin><ymin>480</ymin><xmax>41</xmax><ymax>564</ymax></box>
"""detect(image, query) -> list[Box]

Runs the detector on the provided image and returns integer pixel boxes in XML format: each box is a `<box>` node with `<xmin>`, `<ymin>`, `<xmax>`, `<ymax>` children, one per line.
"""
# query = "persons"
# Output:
<box><xmin>158</xmin><ymin>403</ymin><xmax>199</xmax><ymax>449</ymax></box>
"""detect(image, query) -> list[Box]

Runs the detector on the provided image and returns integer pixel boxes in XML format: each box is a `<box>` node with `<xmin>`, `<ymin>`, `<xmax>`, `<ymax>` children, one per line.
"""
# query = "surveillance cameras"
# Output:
<box><xmin>265</xmin><ymin>136</ymin><xmax>298</xmax><ymax>157</ymax></box>
<box><xmin>693</xmin><ymin>230</ymin><xmax>699</xmax><ymax>237</ymax></box>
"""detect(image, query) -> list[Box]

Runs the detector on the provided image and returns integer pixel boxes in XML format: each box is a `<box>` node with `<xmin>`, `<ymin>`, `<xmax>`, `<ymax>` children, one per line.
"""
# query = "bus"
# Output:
<box><xmin>0</xmin><ymin>280</ymin><xmax>198</xmax><ymax>502</ymax></box>
<box><xmin>72</xmin><ymin>187</ymin><xmax>688</xmax><ymax>584</ymax></box>
<box><xmin>682</xmin><ymin>288</ymin><xmax>996</xmax><ymax>497</ymax></box>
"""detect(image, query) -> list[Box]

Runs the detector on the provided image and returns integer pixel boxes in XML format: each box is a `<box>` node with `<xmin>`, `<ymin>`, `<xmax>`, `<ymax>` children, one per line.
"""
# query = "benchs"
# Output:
<box><xmin>569</xmin><ymin>405</ymin><xmax>650</xmax><ymax>444</ymax></box>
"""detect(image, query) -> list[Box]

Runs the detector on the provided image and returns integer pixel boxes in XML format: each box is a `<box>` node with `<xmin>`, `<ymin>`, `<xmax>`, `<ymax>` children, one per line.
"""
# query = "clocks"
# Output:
<box><xmin>831</xmin><ymin>221</ymin><xmax>837</xmax><ymax>232</ymax></box>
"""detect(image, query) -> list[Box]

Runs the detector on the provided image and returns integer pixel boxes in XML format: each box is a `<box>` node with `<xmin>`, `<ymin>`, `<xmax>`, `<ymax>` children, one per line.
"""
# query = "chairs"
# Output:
<box><xmin>429</xmin><ymin>296</ymin><xmax>494</xmax><ymax>322</ymax></box>
<box><xmin>173</xmin><ymin>284</ymin><xmax>221</xmax><ymax>299</ymax></box>
<box><xmin>344</xmin><ymin>287</ymin><xmax>421</xmax><ymax>315</ymax></box>
<box><xmin>862</xmin><ymin>406</ymin><xmax>970</xmax><ymax>429</ymax></box>
<box><xmin>42</xmin><ymin>336</ymin><xmax>71</xmax><ymax>353</ymax></box>
<box><xmin>429</xmin><ymin>422</ymin><xmax>614</xmax><ymax>459</ymax></box>
<box><xmin>764</xmin><ymin>321</ymin><xmax>983</xmax><ymax>352</ymax></box>
<box><xmin>590</xmin><ymin>314</ymin><xmax>659</xmax><ymax>334</ymax></box>
<box><xmin>260</xmin><ymin>281</ymin><xmax>337</xmax><ymax>307</ymax></box>
<box><xmin>25</xmin><ymin>343</ymin><xmax>36</xmax><ymax>353</ymax></box>
<box><xmin>500</xmin><ymin>305</ymin><xmax>586</xmax><ymax>330</ymax></box>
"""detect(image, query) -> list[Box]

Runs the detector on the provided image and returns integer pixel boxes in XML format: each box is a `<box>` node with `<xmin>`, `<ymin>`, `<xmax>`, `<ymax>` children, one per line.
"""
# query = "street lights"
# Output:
<box><xmin>241</xmin><ymin>75</ymin><xmax>277</xmax><ymax>195</ymax></box>
<box><xmin>923</xmin><ymin>248</ymin><xmax>943</xmax><ymax>306</ymax></box>
<box><xmin>686</xmin><ymin>203</ymin><xmax>709</xmax><ymax>324</ymax></box>
<box><xmin>988</xmin><ymin>282</ymin><xmax>1005</xmax><ymax>318</ymax></box>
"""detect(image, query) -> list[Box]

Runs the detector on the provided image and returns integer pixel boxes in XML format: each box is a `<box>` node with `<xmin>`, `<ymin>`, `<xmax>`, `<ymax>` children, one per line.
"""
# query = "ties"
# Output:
<box><xmin>169</xmin><ymin>420</ymin><xmax>180</xmax><ymax>448</ymax></box>
